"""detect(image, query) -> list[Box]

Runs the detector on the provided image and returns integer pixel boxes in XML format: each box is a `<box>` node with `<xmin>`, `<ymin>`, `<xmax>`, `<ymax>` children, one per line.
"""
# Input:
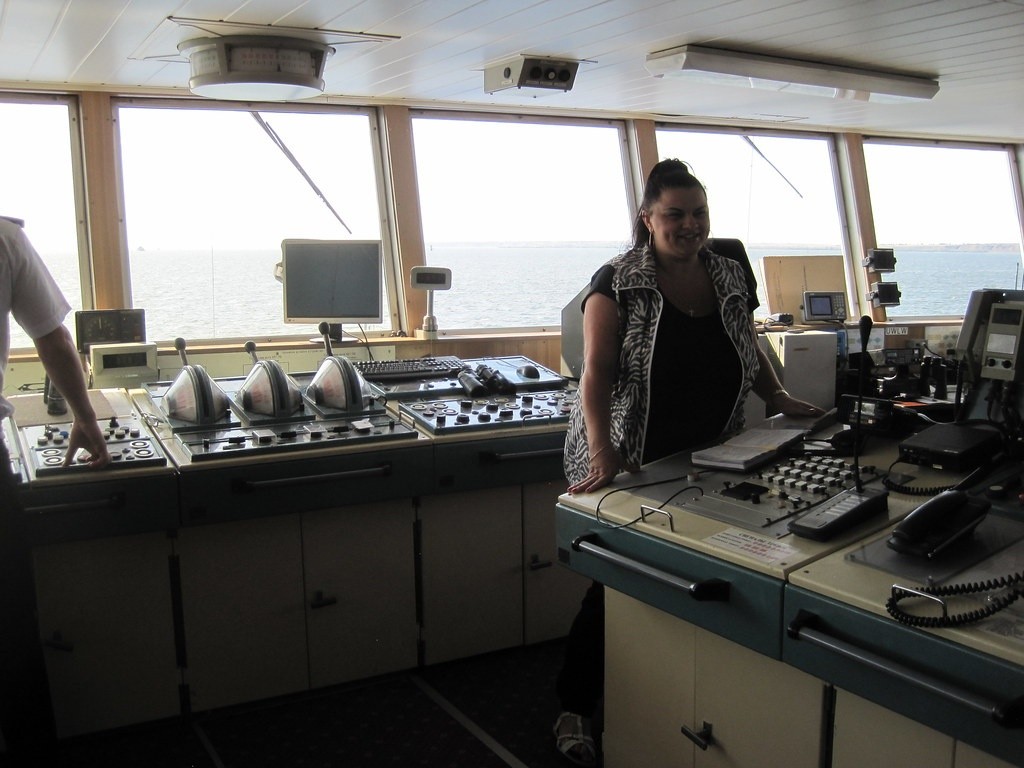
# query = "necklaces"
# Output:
<box><xmin>687</xmin><ymin>306</ymin><xmax>695</xmax><ymax>317</ymax></box>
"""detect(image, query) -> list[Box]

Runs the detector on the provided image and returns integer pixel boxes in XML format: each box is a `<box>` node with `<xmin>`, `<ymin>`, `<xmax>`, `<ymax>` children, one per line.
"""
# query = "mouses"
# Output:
<box><xmin>517</xmin><ymin>366</ymin><xmax>539</xmax><ymax>378</ymax></box>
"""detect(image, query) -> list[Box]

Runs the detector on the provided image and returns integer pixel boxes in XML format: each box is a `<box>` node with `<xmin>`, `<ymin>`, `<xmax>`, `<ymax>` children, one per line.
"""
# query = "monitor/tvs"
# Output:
<box><xmin>282</xmin><ymin>239</ymin><xmax>383</xmax><ymax>324</ymax></box>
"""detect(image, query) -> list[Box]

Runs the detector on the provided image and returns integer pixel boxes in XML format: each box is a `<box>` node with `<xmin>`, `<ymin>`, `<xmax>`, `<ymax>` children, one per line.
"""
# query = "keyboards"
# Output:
<box><xmin>352</xmin><ymin>355</ymin><xmax>469</xmax><ymax>381</ymax></box>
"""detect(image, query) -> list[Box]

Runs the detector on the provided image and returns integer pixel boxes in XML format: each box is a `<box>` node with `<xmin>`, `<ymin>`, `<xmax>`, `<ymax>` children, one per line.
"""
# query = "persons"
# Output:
<box><xmin>543</xmin><ymin>161</ymin><xmax>828</xmax><ymax>768</ymax></box>
<box><xmin>0</xmin><ymin>218</ymin><xmax>113</xmax><ymax>768</ymax></box>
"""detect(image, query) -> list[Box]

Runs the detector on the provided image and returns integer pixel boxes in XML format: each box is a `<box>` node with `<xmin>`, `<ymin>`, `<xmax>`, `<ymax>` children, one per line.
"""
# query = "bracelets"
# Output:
<box><xmin>771</xmin><ymin>390</ymin><xmax>789</xmax><ymax>407</ymax></box>
<box><xmin>589</xmin><ymin>443</ymin><xmax>611</xmax><ymax>462</ymax></box>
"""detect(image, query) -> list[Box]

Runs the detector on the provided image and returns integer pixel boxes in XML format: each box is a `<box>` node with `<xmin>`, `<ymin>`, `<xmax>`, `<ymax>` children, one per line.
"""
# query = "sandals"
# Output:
<box><xmin>552</xmin><ymin>712</ymin><xmax>597</xmax><ymax>764</ymax></box>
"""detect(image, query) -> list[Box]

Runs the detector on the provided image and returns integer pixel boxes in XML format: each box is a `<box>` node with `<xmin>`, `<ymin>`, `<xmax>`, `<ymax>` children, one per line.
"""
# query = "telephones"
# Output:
<box><xmin>884</xmin><ymin>487</ymin><xmax>991</xmax><ymax>560</ymax></box>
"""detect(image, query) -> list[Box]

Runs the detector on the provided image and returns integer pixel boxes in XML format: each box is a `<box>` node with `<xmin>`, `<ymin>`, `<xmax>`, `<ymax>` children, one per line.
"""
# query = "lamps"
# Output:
<box><xmin>646</xmin><ymin>45</ymin><xmax>940</xmax><ymax>106</ymax></box>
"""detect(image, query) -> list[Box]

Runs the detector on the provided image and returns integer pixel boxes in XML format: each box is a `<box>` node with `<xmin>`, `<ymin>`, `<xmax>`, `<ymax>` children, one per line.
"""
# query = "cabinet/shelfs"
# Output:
<box><xmin>181</xmin><ymin>498</ymin><xmax>422</xmax><ymax>721</ymax></box>
<box><xmin>424</xmin><ymin>477</ymin><xmax>600</xmax><ymax>674</ymax></box>
<box><xmin>831</xmin><ymin>682</ymin><xmax>1020</xmax><ymax>768</ymax></box>
<box><xmin>603</xmin><ymin>585</ymin><xmax>830</xmax><ymax>768</ymax></box>
<box><xmin>28</xmin><ymin>530</ymin><xmax>183</xmax><ymax>742</ymax></box>
